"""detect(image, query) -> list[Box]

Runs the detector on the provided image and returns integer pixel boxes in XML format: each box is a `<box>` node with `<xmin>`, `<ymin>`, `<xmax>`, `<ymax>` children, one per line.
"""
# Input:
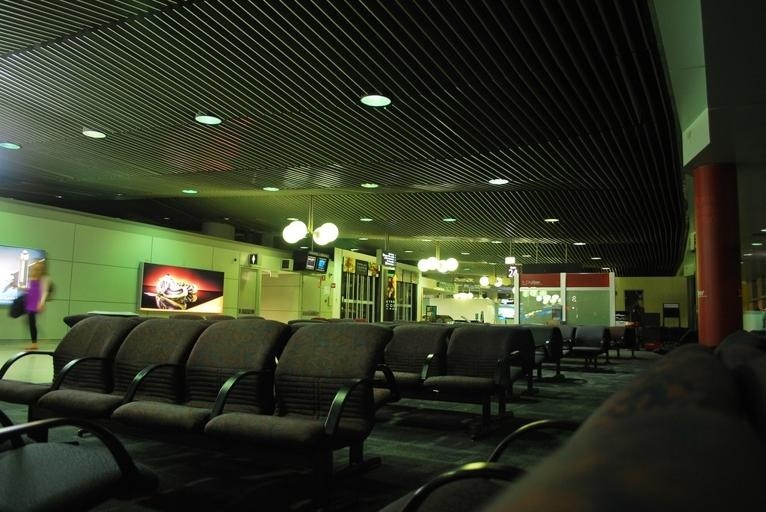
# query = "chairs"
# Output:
<box><xmin>1</xmin><ymin>312</ymin><xmax>766</xmax><ymax>511</ymax></box>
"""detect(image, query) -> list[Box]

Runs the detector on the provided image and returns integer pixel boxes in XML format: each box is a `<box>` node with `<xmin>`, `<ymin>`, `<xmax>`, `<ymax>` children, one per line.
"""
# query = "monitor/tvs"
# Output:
<box><xmin>293</xmin><ymin>249</ymin><xmax>315</xmax><ymax>272</ymax></box>
<box><xmin>316</xmin><ymin>254</ymin><xmax>329</xmax><ymax>272</ymax></box>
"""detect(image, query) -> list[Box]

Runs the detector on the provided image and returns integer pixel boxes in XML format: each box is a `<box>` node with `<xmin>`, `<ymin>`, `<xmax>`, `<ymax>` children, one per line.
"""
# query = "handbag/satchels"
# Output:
<box><xmin>11</xmin><ymin>296</ymin><xmax>26</xmax><ymax>318</ymax></box>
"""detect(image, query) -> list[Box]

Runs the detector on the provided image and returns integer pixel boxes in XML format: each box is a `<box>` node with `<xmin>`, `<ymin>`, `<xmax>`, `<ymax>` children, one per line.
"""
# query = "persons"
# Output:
<box><xmin>22</xmin><ymin>262</ymin><xmax>49</xmax><ymax>351</ymax></box>
